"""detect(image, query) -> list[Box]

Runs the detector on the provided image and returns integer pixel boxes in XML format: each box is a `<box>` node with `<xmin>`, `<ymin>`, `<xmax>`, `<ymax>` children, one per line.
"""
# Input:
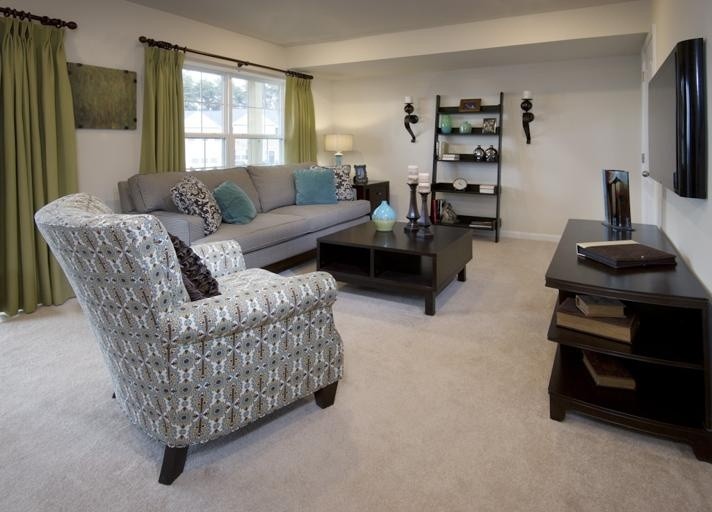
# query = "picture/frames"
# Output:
<box><xmin>459</xmin><ymin>98</ymin><xmax>481</xmax><ymax>113</ymax></box>
<box><xmin>353</xmin><ymin>165</ymin><xmax>368</xmax><ymax>183</ymax></box>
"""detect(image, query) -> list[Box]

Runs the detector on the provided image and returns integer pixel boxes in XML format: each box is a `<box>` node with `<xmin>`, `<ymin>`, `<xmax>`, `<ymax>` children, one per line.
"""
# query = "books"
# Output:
<box><xmin>469</xmin><ymin>224</ymin><xmax>493</xmax><ymax>229</ymax></box>
<box><xmin>479</xmin><ymin>188</ymin><xmax>495</xmax><ymax>194</ymax></box>
<box><xmin>555</xmin><ymin>297</ymin><xmax>641</xmax><ymax>344</ymax></box>
<box><xmin>471</xmin><ymin>221</ymin><xmax>492</xmax><ymax>226</ymax></box>
<box><xmin>582</xmin><ymin>351</ymin><xmax>636</xmax><ymax>391</ymax></box>
<box><xmin>436</xmin><ymin>141</ymin><xmax>460</xmax><ymax>161</ymax></box>
<box><xmin>479</xmin><ymin>184</ymin><xmax>496</xmax><ymax>189</ymax></box>
<box><xmin>433</xmin><ymin>199</ymin><xmax>446</xmax><ymax>224</ymax></box>
<box><xmin>575</xmin><ymin>294</ymin><xmax>627</xmax><ymax>319</ymax></box>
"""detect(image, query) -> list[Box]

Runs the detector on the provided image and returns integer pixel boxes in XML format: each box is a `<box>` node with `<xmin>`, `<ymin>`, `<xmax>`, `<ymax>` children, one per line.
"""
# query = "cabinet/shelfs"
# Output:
<box><xmin>543</xmin><ymin>218</ymin><xmax>711</xmax><ymax>460</ymax></box>
<box><xmin>428</xmin><ymin>92</ymin><xmax>504</xmax><ymax>244</ymax></box>
<box><xmin>350</xmin><ymin>180</ymin><xmax>390</xmax><ymax>219</ymax></box>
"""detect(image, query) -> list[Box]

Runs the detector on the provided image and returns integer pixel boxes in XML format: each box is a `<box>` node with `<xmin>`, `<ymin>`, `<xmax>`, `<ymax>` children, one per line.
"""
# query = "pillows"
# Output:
<box><xmin>309</xmin><ymin>165</ymin><xmax>354</xmax><ymax>200</ymax></box>
<box><xmin>245</xmin><ymin>162</ymin><xmax>317</xmax><ymax>213</ymax></box>
<box><xmin>166</xmin><ymin>175</ymin><xmax>222</xmax><ymax>236</ymax></box>
<box><xmin>212</xmin><ymin>180</ymin><xmax>257</xmax><ymax>224</ymax></box>
<box><xmin>294</xmin><ymin>168</ymin><xmax>337</xmax><ymax>206</ymax></box>
<box><xmin>125</xmin><ymin>167</ymin><xmax>262</xmax><ymax>214</ymax></box>
<box><xmin>166</xmin><ymin>233</ymin><xmax>223</xmax><ymax>301</ymax></box>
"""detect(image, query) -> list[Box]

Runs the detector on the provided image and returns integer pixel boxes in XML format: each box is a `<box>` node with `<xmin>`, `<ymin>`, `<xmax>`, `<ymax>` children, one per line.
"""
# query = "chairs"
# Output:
<box><xmin>33</xmin><ymin>192</ymin><xmax>343</xmax><ymax>486</ymax></box>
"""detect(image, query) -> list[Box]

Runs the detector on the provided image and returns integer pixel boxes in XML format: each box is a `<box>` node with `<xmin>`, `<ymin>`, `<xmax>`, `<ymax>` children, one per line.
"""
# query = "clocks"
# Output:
<box><xmin>452</xmin><ymin>177</ymin><xmax>468</xmax><ymax>190</ymax></box>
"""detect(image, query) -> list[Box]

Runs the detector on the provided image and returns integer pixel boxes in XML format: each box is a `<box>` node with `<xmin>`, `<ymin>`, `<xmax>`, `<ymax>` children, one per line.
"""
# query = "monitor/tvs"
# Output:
<box><xmin>648</xmin><ymin>36</ymin><xmax>708</xmax><ymax>199</ymax></box>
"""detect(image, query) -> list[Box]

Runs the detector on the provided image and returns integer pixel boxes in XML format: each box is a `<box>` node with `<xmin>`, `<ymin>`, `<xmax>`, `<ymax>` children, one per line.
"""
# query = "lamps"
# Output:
<box><xmin>324</xmin><ymin>133</ymin><xmax>353</xmax><ymax>168</ymax></box>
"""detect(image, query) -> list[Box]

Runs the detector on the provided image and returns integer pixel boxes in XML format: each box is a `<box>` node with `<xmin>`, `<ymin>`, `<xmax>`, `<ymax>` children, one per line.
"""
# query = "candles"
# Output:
<box><xmin>523</xmin><ymin>90</ymin><xmax>534</xmax><ymax>99</ymax></box>
<box><xmin>409</xmin><ymin>166</ymin><xmax>419</xmax><ymax>184</ymax></box>
<box><xmin>419</xmin><ymin>173</ymin><xmax>430</xmax><ymax>192</ymax></box>
<box><xmin>406</xmin><ymin>96</ymin><xmax>413</xmax><ymax>103</ymax></box>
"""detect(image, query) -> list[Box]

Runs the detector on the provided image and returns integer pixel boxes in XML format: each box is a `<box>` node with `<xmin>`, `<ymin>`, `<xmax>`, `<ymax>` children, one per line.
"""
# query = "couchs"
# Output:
<box><xmin>116</xmin><ymin>181</ymin><xmax>371</xmax><ymax>270</ymax></box>
